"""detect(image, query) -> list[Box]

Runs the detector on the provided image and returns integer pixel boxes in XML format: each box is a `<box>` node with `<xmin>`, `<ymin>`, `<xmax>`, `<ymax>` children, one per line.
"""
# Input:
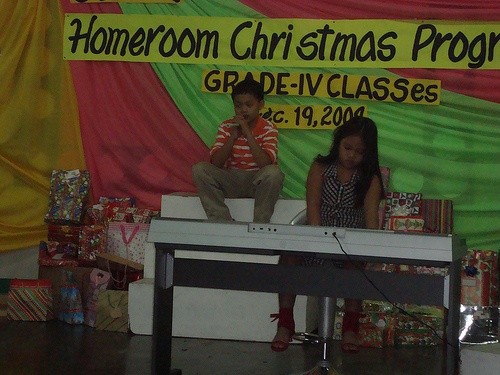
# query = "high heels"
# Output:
<box><xmin>342</xmin><ymin>313</ymin><xmax>360</xmax><ymax>353</ymax></box>
<box><xmin>270</xmin><ymin>308</ymin><xmax>295</xmax><ymax>351</ymax></box>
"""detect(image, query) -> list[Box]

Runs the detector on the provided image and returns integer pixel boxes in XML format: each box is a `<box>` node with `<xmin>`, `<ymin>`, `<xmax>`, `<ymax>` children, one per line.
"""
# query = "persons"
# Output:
<box><xmin>193</xmin><ymin>80</ymin><xmax>285</xmax><ymax>223</ymax></box>
<box><xmin>270</xmin><ymin>116</ymin><xmax>385</xmax><ymax>353</ymax></box>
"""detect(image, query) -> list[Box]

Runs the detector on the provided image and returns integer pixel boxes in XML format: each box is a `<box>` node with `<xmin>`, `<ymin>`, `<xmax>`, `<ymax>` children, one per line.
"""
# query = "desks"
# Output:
<box><xmin>150</xmin><ymin>242</ymin><xmax>463</xmax><ymax>375</ymax></box>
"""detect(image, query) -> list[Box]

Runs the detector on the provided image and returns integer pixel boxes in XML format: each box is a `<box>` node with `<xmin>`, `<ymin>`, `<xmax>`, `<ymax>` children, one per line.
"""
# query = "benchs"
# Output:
<box><xmin>128</xmin><ymin>193</ymin><xmax>318</xmax><ymax>344</ymax></box>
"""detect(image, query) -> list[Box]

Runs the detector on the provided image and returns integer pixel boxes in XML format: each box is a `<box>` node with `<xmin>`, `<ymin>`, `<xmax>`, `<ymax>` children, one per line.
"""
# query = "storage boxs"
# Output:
<box><xmin>332</xmin><ymin>167</ymin><xmax>500</xmax><ymax>347</ymax></box>
<box><xmin>5</xmin><ymin>170</ymin><xmax>160</xmax><ymax>333</ymax></box>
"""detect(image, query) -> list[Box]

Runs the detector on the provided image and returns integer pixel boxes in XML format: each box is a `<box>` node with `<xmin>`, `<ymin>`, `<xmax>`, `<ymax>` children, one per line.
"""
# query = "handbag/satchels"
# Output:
<box><xmin>0</xmin><ymin>168</ymin><xmax>159</xmax><ymax>335</ymax></box>
